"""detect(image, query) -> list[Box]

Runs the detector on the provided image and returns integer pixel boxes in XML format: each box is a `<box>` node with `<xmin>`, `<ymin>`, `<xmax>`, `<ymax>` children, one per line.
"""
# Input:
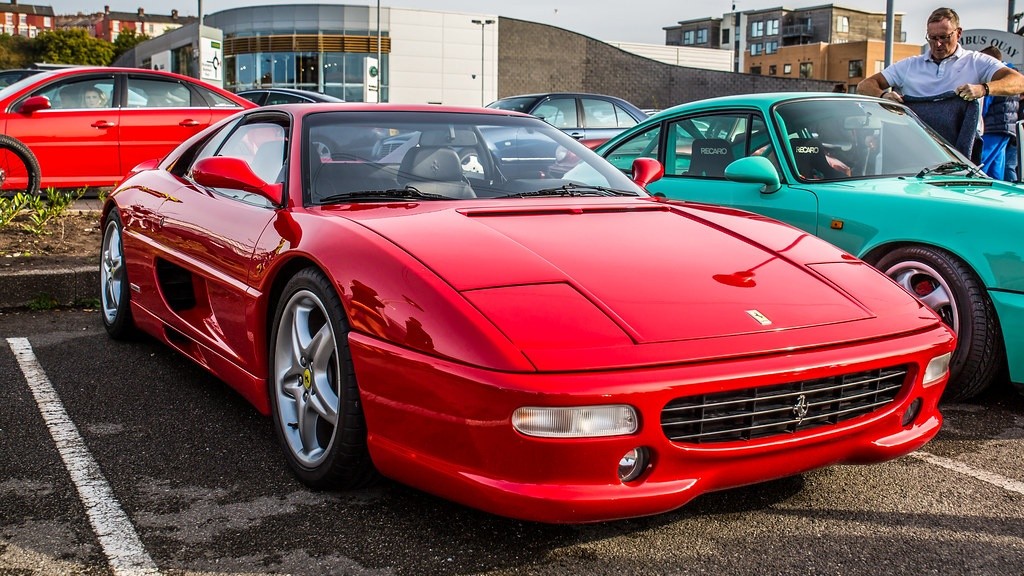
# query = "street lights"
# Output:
<box><xmin>471</xmin><ymin>19</ymin><xmax>497</xmax><ymax>108</ymax></box>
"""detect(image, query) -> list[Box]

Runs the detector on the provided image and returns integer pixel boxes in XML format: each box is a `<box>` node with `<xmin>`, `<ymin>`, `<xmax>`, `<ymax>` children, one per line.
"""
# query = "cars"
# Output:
<box><xmin>0</xmin><ymin>62</ymin><xmax>148</xmax><ymax>107</ymax></box>
<box><xmin>371</xmin><ymin>89</ymin><xmax>733</xmax><ymax>183</ymax></box>
<box><xmin>232</xmin><ymin>88</ymin><xmax>391</xmax><ymax>162</ymax></box>
<box><xmin>0</xmin><ymin>66</ymin><xmax>292</xmax><ymax>201</ymax></box>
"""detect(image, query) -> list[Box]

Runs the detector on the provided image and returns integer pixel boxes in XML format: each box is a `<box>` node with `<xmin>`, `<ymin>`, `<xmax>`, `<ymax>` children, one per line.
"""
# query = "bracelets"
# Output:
<box><xmin>981</xmin><ymin>82</ymin><xmax>989</xmax><ymax>96</ymax></box>
<box><xmin>880</xmin><ymin>91</ymin><xmax>889</xmax><ymax>98</ymax></box>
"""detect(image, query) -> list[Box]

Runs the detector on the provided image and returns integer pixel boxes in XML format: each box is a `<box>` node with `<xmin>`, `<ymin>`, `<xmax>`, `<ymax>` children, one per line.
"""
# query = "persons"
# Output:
<box><xmin>833</xmin><ymin>82</ymin><xmax>849</xmax><ymax>94</ymax></box>
<box><xmin>80</xmin><ymin>87</ymin><xmax>109</xmax><ymax>108</ymax></box>
<box><xmin>59</xmin><ymin>86</ymin><xmax>85</xmax><ymax>109</ymax></box>
<box><xmin>856</xmin><ymin>8</ymin><xmax>1023</xmax><ymax>184</ymax></box>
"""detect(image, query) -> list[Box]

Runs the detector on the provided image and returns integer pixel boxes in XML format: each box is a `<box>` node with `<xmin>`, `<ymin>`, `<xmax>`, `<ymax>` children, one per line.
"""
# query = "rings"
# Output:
<box><xmin>965</xmin><ymin>96</ymin><xmax>969</xmax><ymax>99</ymax></box>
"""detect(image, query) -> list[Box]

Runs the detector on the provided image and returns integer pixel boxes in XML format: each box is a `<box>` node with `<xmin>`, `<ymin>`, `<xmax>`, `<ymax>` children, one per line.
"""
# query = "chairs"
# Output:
<box><xmin>682</xmin><ymin>138</ymin><xmax>734</xmax><ymax>177</ymax></box>
<box><xmin>790</xmin><ymin>137</ymin><xmax>849</xmax><ymax>181</ymax></box>
<box><xmin>397</xmin><ymin>145</ymin><xmax>477</xmax><ymax>199</ymax></box>
<box><xmin>246</xmin><ymin>141</ymin><xmax>335</xmax><ymax>205</ymax></box>
<box><xmin>60</xmin><ymin>86</ymin><xmax>80</xmax><ymax>108</ymax></box>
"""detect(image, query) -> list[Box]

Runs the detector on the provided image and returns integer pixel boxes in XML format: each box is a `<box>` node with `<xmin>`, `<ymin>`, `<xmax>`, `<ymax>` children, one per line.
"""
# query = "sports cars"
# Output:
<box><xmin>555</xmin><ymin>91</ymin><xmax>1024</xmax><ymax>406</ymax></box>
<box><xmin>93</xmin><ymin>100</ymin><xmax>959</xmax><ymax>527</ymax></box>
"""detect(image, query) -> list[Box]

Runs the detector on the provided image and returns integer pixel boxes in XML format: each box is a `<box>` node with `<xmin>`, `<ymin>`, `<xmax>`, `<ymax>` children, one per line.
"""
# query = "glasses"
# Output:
<box><xmin>926</xmin><ymin>28</ymin><xmax>958</xmax><ymax>44</ymax></box>
